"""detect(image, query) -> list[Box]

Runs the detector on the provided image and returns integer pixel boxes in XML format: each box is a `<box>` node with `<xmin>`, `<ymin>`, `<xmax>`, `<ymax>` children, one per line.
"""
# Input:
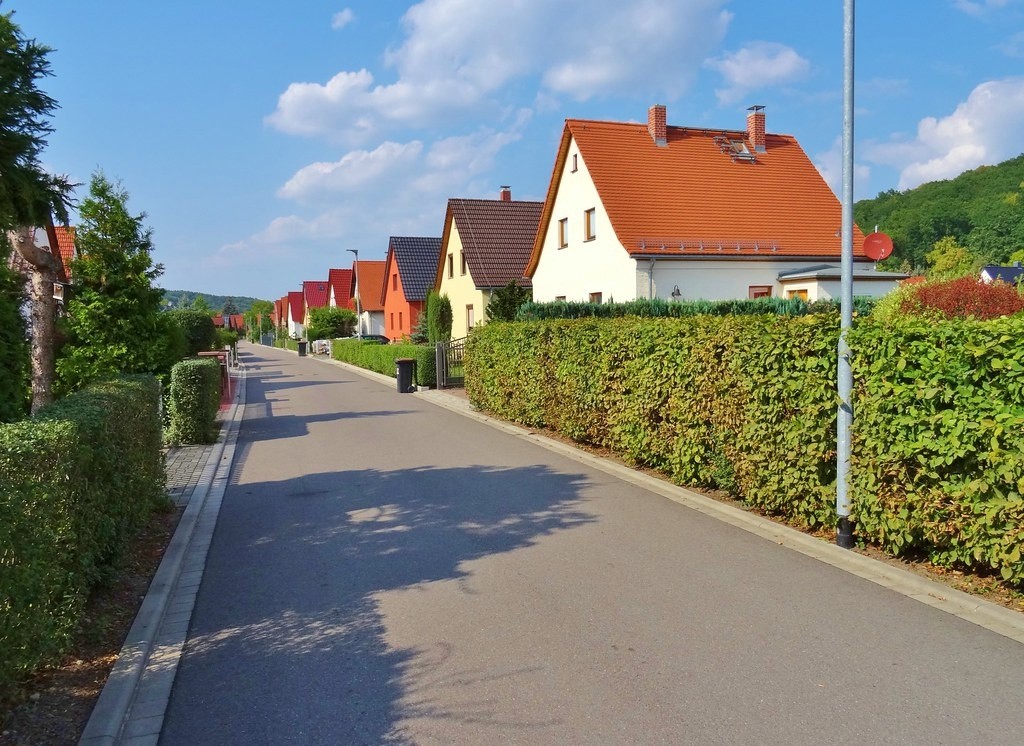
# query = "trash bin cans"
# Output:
<box><xmin>297</xmin><ymin>341</ymin><xmax>308</xmax><ymax>357</ymax></box>
<box><xmin>395</xmin><ymin>358</ymin><xmax>417</xmax><ymax>394</ymax></box>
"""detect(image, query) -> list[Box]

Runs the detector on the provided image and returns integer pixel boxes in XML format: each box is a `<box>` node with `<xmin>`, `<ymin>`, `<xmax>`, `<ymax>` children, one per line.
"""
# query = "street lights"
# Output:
<box><xmin>346</xmin><ymin>249</ymin><xmax>361</xmax><ymax>340</ymax></box>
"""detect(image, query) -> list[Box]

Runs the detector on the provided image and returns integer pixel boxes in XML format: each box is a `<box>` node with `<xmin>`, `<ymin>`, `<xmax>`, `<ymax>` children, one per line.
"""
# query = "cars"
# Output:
<box><xmin>350</xmin><ymin>335</ymin><xmax>390</xmax><ymax>344</ymax></box>
